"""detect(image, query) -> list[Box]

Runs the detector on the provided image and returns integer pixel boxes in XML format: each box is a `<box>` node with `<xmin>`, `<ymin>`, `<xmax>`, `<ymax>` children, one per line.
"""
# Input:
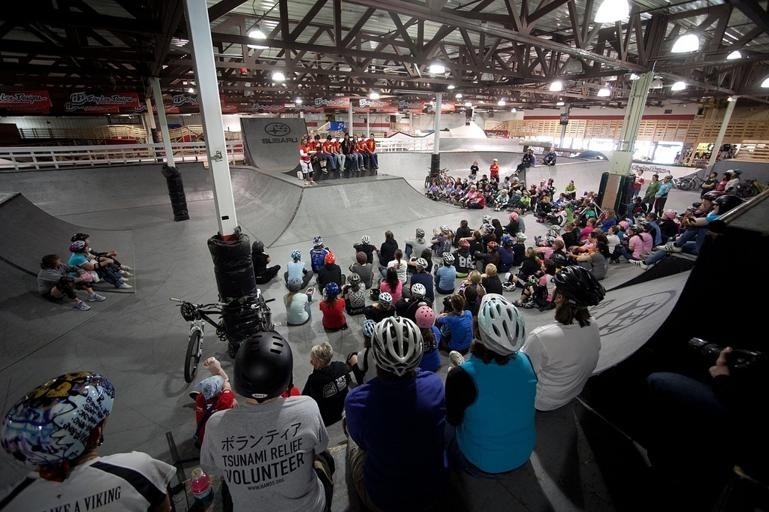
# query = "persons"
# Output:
<box><xmin>299</xmin><ymin>133</ymin><xmax>378</xmax><ymax>184</ymax></box>
<box><xmin>302</xmin><ymin>344</ymin><xmax>357</xmax><ymax>426</ymax></box>
<box><xmin>515</xmin><ymin>266</ymin><xmax>606</xmax><ymax>413</ymax></box>
<box><xmin>445</xmin><ymin>293</ymin><xmax>537</xmax><ymax>477</ymax></box>
<box><xmin>37</xmin><ymin>232</ymin><xmax>132</xmax><ymax>310</ymax></box>
<box><xmin>346</xmin><ymin>319</ymin><xmax>379</xmax><ymax>384</ymax></box>
<box><xmin>281</xmin><ymin>387</ymin><xmax>299</xmax><ymax>400</ymax></box>
<box><xmin>251</xmin><ymin>240</ymin><xmax>281</xmax><ymax>284</ymax></box>
<box><xmin>645</xmin><ymin>346</ymin><xmax>769</xmax><ymax>512</ymax></box>
<box><xmin>1</xmin><ymin>370</ymin><xmax>176</xmax><ymax>512</ymax></box>
<box><xmin>196</xmin><ymin>357</ymin><xmax>239</xmax><ymax>444</ymax></box>
<box><xmin>284</xmin><ymin>141</ymin><xmax>763</xmax><ymax>374</ymax></box>
<box><xmin>346</xmin><ymin>316</ymin><xmax>450</xmax><ymax>512</ymax></box>
<box><xmin>200</xmin><ymin>330</ymin><xmax>336</xmax><ymax>511</ymax></box>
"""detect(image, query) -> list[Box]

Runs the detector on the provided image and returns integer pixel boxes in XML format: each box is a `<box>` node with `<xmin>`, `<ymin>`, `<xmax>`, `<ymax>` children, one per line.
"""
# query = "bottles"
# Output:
<box><xmin>190</xmin><ymin>467</ymin><xmax>214</xmax><ymax>505</ymax></box>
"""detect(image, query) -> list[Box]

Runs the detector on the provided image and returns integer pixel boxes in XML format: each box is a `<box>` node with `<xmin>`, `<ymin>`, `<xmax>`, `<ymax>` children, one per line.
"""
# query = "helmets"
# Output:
<box><xmin>1</xmin><ymin>371</ymin><xmax>115</xmax><ymax>464</ymax></box>
<box><xmin>70</xmin><ymin>232</ymin><xmax>88</xmax><ymax>253</ymax></box>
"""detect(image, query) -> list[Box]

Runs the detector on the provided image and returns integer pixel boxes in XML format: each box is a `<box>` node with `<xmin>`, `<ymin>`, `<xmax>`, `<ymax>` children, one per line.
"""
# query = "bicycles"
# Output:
<box><xmin>682</xmin><ymin>173</ymin><xmax>708</xmax><ymax>191</ymax></box>
<box><xmin>169</xmin><ymin>295</ymin><xmax>275</xmax><ymax>384</ymax></box>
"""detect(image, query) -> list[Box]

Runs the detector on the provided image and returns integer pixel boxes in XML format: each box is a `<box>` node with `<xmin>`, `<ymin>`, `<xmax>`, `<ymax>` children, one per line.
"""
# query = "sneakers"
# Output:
<box><xmin>189</xmin><ymin>391</ymin><xmax>200</xmax><ymax>400</ymax></box>
<box><xmin>73</xmin><ymin>266</ymin><xmax>133</xmax><ymax>311</ymax></box>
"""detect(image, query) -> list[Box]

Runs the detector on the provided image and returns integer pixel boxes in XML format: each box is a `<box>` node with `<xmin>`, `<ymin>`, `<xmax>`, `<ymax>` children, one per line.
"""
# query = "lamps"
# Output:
<box><xmin>593</xmin><ymin>0</ymin><xmax>631</xmax><ymax>24</ymax></box>
<box><xmin>246</xmin><ymin>17</ymin><xmax>270</xmax><ymax>50</ymax></box>
<box><xmin>669</xmin><ymin>26</ymin><xmax>700</xmax><ymax>54</ymax></box>
